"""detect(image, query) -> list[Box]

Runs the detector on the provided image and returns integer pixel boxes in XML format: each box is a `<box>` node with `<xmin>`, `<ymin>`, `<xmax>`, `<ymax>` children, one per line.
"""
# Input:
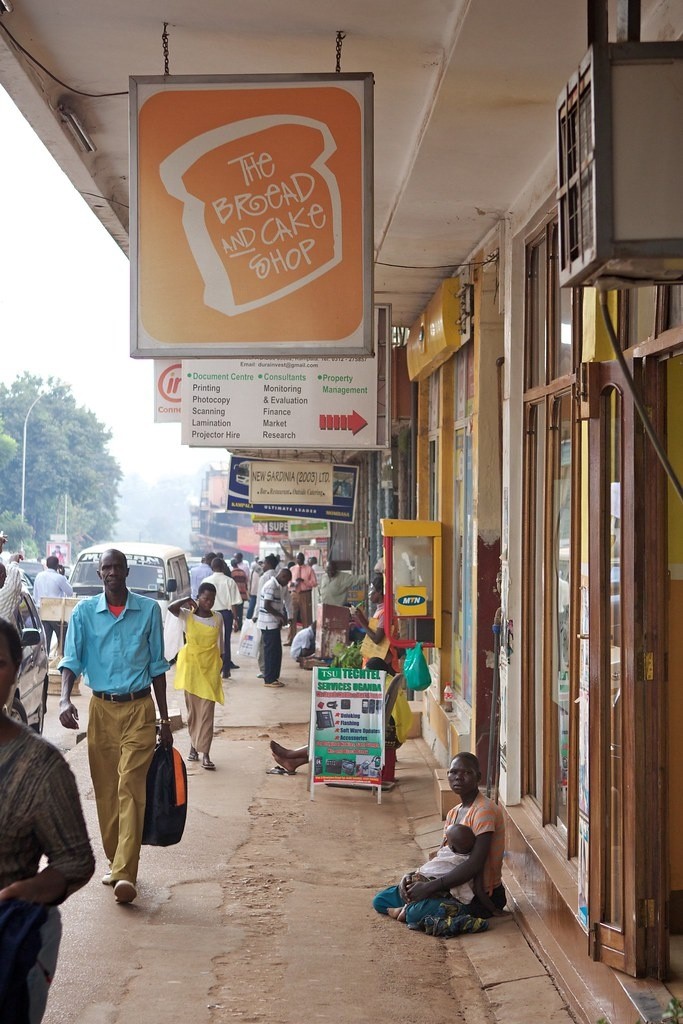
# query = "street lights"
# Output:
<box><xmin>19</xmin><ymin>380</ymin><xmax>74</xmax><ymax>553</ymax></box>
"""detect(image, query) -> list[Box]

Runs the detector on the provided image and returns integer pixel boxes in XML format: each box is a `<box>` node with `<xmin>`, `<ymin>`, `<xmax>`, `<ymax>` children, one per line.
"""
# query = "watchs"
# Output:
<box><xmin>159</xmin><ymin>719</ymin><xmax>172</xmax><ymax>725</ymax></box>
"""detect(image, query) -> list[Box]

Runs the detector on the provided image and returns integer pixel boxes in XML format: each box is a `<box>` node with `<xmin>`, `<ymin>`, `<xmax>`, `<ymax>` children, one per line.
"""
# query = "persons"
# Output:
<box><xmin>58</xmin><ymin>548</ymin><xmax>173</xmax><ymax>903</ymax></box>
<box><xmin>320</xmin><ymin>561</ymin><xmax>353</xmax><ymax>606</ymax></box>
<box><xmin>0</xmin><ymin>617</ymin><xmax>96</xmax><ymax>1024</ymax></box>
<box><xmin>269</xmin><ymin>657</ymin><xmax>413</xmax><ymax>772</ymax></box>
<box><xmin>190</xmin><ymin>551</ymin><xmax>318</xmax><ymax>679</ymax></box>
<box><xmin>52</xmin><ymin>546</ymin><xmax>64</xmax><ymax>565</ymax></box>
<box><xmin>33</xmin><ymin>556</ymin><xmax>73</xmax><ymax>657</ymax></box>
<box><xmin>350</xmin><ymin>577</ymin><xmax>398</xmax><ymax>664</ymax></box>
<box><xmin>168</xmin><ymin>582</ymin><xmax>225</xmax><ymax>769</ymax></box>
<box><xmin>259</xmin><ymin>569</ymin><xmax>292</xmax><ymax>687</ymax></box>
<box><xmin>291</xmin><ymin>621</ymin><xmax>317</xmax><ymax>662</ymax></box>
<box><xmin>372</xmin><ymin>753</ymin><xmax>506</xmax><ymax>923</ymax></box>
<box><xmin>0</xmin><ymin>531</ymin><xmax>25</xmax><ymax>626</ymax></box>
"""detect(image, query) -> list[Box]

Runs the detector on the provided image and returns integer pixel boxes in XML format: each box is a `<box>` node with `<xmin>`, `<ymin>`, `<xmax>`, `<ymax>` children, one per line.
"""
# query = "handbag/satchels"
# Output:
<box><xmin>246</xmin><ymin>597</ymin><xmax>255</xmax><ymax>619</ymax></box>
<box><xmin>237</xmin><ymin>618</ymin><xmax>262</xmax><ymax>659</ymax></box>
<box><xmin>404</xmin><ymin>642</ymin><xmax>432</xmax><ymax>691</ymax></box>
<box><xmin>140</xmin><ymin>743</ymin><xmax>188</xmax><ymax>846</ymax></box>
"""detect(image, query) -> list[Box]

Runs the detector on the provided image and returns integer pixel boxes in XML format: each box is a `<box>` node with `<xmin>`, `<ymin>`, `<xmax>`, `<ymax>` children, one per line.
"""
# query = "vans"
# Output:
<box><xmin>67</xmin><ymin>540</ymin><xmax>193</xmax><ymax>666</ymax></box>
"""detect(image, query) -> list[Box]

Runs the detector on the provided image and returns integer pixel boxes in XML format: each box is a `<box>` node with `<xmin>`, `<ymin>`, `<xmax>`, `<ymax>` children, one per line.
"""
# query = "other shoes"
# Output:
<box><xmin>264</xmin><ymin>679</ymin><xmax>284</xmax><ymax>687</ymax></box>
<box><xmin>223</xmin><ymin>673</ymin><xmax>231</xmax><ymax>678</ymax></box>
<box><xmin>113</xmin><ymin>880</ymin><xmax>137</xmax><ymax>903</ymax></box>
<box><xmin>283</xmin><ymin>642</ymin><xmax>291</xmax><ymax>646</ymax></box>
<box><xmin>257</xmin><ymin>673</ymin><xmax>266</xmax><ymax>678</ymax></box>
<box><xmin>102</xmin><ymin>869</ymin><xmax>113</xmax><ymax>885</ymax></box>
<box><xmin>230</xmin><ymin>661</ymin><xmax>240</xmax><ymax>669</ymax></box>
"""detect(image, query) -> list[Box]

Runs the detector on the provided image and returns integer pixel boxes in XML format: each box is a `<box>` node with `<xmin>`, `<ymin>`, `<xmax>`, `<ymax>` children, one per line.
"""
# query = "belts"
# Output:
<box><xmin>92</xmin><ymin>687</ymin><xmax>151</xmax><ymax>702</ymax></box>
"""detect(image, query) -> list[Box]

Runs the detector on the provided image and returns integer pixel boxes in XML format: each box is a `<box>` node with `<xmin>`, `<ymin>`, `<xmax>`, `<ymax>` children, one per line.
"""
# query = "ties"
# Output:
<box><xmin>296</xmin><ymin>567</ymin><xmax>301</xmax><ymax>593</ymax></box>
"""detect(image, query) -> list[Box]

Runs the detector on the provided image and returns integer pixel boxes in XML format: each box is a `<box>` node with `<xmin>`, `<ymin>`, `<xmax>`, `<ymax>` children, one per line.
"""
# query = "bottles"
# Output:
<box><xmin>355</xmin><ymin>598</ymin><xmax>366</xmax><ymax>615</ymax></box>
<box><xmin>444</xmin><ymin>682</ymin><xmax>453</xmax><ymax>711</ymax></box>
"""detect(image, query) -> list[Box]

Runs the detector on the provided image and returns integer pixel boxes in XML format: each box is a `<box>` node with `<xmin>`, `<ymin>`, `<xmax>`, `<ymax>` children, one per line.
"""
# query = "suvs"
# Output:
<box><xmin>7</xmin><ymin>584</ymin><xmax>50</xmax><ymax>736</ymax></box>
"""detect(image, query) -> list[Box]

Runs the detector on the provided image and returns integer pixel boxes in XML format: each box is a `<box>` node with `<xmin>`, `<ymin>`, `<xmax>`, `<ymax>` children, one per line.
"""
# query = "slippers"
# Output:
<box><xmin>187</xmin><ymin>752</ymin><xmax>198</xmax><ymax>761</ymax></box>
<box><xmin>267</xmin><ymin>765</ymin><xmax>297</xmax><ymax>775</ymax></box>
<box><xmin>202</xmin><ymin>759</ymin><xmax>215</xmax><ymax>769</ymax></box>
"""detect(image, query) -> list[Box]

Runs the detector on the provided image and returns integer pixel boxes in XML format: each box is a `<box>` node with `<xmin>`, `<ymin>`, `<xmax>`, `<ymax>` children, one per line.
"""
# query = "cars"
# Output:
<box><xmin>16</xmin><ymin>558</ymin><xmax>72</xmax><ymax>612</ymax></box>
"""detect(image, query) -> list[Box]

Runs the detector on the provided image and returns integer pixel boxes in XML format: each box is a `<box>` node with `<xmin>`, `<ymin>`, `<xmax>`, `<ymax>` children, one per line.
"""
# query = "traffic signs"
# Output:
<box><xmin>177</xmin><ymin>299</ymin><xmax>393</xmax><ymax>453</ymax></box>
